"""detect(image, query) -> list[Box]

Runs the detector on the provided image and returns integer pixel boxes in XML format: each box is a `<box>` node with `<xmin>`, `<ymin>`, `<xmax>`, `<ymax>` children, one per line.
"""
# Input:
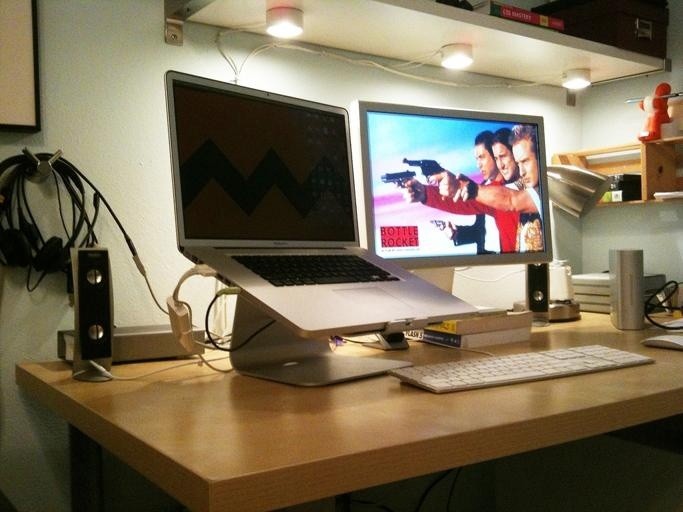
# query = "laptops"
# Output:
<box><xmin>164</xmin><ymin>69</ymin><xmax>480</xmax><ymax>338</ymax></box>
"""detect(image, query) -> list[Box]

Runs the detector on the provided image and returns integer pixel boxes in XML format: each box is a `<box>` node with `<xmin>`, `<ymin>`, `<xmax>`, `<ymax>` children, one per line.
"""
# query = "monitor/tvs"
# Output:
<box><xmin>350</xmin><ymin>99</ymin><xmax>553</xmax><ymax>317</ymax></box>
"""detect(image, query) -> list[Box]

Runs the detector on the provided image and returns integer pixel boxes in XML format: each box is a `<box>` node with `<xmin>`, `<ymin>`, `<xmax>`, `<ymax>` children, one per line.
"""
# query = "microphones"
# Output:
<box><xmin>77</xmin><ymin>192</ymin><xmax>99</xmax><ymax>247</ymax></box>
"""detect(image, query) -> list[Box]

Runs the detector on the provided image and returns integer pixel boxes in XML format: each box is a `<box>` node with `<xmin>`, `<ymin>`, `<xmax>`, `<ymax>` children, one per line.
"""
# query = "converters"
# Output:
<box><xmin>57</xmin><ymin>323</ymin><xmax>205</xmax><ymax>364</ymax></box>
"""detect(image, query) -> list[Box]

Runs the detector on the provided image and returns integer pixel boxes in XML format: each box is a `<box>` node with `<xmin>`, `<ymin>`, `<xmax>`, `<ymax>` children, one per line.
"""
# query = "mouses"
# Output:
<box><xmin>641</xmin><ymin>335</ymin><xmax>683</xmax><ymax>350</ymax></box>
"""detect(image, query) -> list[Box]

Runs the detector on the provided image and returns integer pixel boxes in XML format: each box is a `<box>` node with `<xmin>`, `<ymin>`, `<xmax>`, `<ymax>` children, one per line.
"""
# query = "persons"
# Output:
<box><xmin>396</xmin><ymin>123</ymin><xmax>544</xmax><ymax>254</ymax></box>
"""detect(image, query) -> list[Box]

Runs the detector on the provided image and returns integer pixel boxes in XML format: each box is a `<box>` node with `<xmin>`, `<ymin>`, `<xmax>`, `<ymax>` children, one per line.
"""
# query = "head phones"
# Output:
<box><xmin>0</xmin><ymin>154</ymin><xmax>84</xmax><ymax>275</ymax></box>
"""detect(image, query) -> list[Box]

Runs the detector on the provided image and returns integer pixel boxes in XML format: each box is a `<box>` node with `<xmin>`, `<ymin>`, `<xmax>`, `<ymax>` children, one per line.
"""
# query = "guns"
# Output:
<box><xmin>403</xmin><ymin>159</ymin><xmax>442</xmax><ymax>176</ymax></box>
<box><xmin>431</xmin><ymin>220</ymin><xmax>456</xmax><ymax>239</ymax></box>
<box><xmin>381</xmin><ymin>172</ymin><xmax>416</xmax><ymax>188</ymax></box>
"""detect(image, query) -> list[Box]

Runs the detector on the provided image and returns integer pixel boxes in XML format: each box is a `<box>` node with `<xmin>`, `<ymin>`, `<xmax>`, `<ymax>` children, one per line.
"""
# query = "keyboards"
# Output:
<box><xmin>387</xmin><ymin>345</ymin><xmax>654</xmax><ymax>394</ymax></box>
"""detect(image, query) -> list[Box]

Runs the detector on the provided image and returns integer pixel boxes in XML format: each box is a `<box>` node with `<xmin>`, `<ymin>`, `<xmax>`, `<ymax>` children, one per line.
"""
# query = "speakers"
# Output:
<box><xmin>70</xmin><ymin>247</ymin><xmax>114</xmax><ymax>382</ymax></box>
<box><xmin>525</xmin><ymin>263</ymin><xmax>552</xmax><ymax>327</ymax></box>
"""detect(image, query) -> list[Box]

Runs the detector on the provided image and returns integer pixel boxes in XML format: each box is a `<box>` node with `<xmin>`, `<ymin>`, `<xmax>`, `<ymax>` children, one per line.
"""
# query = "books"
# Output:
<box><xmin>473</xmin><ymin>0</ymin><xmax>565</xmax><ymax>32</ymax></box>
<box><xmin>600</xmin><ymin>174</ymin><xmax>641</xmax><ymax>204</ymax></box>
<box><xmin>424</xmin><ymin>311</ymin><xmax>533</xmax><ymax>335</ymax></box>
<box><xmin>403</xmin><ymin>328</ymin><xmax>531</xmax><ymax>349</ymax></box>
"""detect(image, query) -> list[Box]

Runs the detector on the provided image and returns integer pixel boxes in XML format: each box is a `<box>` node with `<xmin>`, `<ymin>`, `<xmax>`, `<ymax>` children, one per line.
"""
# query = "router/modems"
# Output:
<box><xmin>609</xmin><ymin>247</ymin><xmax>644</xmax><ymax>330</ymax></box>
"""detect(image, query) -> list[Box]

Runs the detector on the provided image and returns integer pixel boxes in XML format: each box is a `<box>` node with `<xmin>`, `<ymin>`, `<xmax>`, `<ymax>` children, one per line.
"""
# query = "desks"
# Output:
<box><xmin>13</xmin><ymin>305</ymin><xmax>683</xmax><ymax>512</ymax></box>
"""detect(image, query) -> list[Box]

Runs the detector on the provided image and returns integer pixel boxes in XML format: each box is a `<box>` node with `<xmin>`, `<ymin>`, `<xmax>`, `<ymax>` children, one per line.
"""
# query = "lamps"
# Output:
<box><xmin>540</xmin><ymin>167</ymin><xmax>614</xmax><ymax>324</ymax></box>
<box><xmin>265</xmin><ymin>9</ymin><xmax>307</xmax><ymax>40</ymax></box>
<box><xmin>560</xmin><ymin>66</ymin><xmax>593</xmax><ymax>92</ymax></box>
<box><xmin>440</xmin><ymin>44</ymin><xmax>475</xmax><ymax>70</ymax></box>
